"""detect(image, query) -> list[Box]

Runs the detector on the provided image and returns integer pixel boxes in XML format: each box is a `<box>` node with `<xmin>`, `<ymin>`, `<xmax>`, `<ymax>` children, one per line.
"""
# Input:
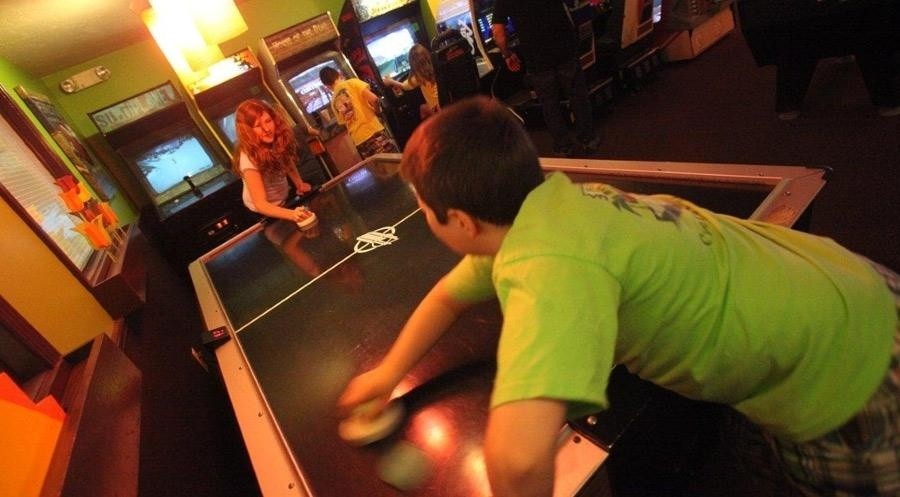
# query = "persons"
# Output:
<box><xmin>318</xmin><ymin>66</ymin><xmax>398</xmax><ymax>161</ymax></box>
<box><xmin>335</xmin><ymin>94</ymin><xmax>900</xmax><ymax>497</ymax></box>
<box><xmin>229</xmin><ymin>96</ymin><xmax>312</xmax><ymax>227</ymax></box>
<box><xmin>382</xmin><ymin>42</ymin><xmax>440</xmax><ymax>124</ymax></box>
<box><xmin>265</xmin><ymin>191</ymin><xmax>366</xmax><ymax>294</ymax></box>
<box><xmin>492</xmin><ymin>0</ymin><xmax>601</xmax><ymax>158</ymax></box>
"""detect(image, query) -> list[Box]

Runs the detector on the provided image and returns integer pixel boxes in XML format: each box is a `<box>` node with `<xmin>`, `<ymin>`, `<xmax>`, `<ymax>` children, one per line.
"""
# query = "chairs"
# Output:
<box><xmin>575</xmin><ymin>0</ymin><xmax>655</xmax><ymax>70</ymax></box>
<box><xmin>430</xmin><ymin>29</ymin><xmax>481</xmax><ymax>108</ymax></box>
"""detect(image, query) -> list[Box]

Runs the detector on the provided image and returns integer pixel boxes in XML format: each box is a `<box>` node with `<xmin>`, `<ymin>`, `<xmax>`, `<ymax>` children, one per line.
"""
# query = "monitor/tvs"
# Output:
<box><xmin>652</xmin><ymin>0</ymin><xmax>662</xmax><ymax>23</ymax></box>
<box><xmin>216</xmin><ymin>98</ymin><xmax>275</xmax><ymax>147</ymax></box>
<box><xmin>477</xmin><ymin>11</ymin><xmax>517</xmax><ymax>46</ymax></box>
<box><xmin>366</xmin><ymin>27</ymin><xmax>419</xmax><ymax>82</ymax></box>
<box><xmin>288</xmin><ymin>60</ymin><xmax>347</xmax><ymax>115</ymax></box>
<box><xmin>135</xmin><ymin>132</ymin><xmax>214</xmax><ymax>195</ymax></box>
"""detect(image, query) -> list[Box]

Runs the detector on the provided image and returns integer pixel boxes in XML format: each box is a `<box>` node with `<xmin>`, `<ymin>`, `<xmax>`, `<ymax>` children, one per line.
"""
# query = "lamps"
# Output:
<box><xmin>141</xmin><ymin>0</ymin><xmax>249</xmax><ymax>87</ymax></box>
<box><xmin>60</xmin><ymin>64</ymin><xmax>112</xmax><ymax>93</ymax></box>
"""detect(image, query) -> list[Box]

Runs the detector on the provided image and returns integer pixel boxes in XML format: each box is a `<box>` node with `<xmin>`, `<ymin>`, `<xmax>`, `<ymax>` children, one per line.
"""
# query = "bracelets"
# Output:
<box><xmin>500</xmin><ymin>50</ymin><xmax>512</xmax><ymax>61</ymax></box>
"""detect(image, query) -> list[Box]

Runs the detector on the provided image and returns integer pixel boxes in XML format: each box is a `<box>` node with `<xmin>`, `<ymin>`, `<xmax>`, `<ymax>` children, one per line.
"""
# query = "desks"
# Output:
<box><xmin>188</xmin><ymin>152</ymin><xmax>838</xmax><ymax>497</ymax></box>
